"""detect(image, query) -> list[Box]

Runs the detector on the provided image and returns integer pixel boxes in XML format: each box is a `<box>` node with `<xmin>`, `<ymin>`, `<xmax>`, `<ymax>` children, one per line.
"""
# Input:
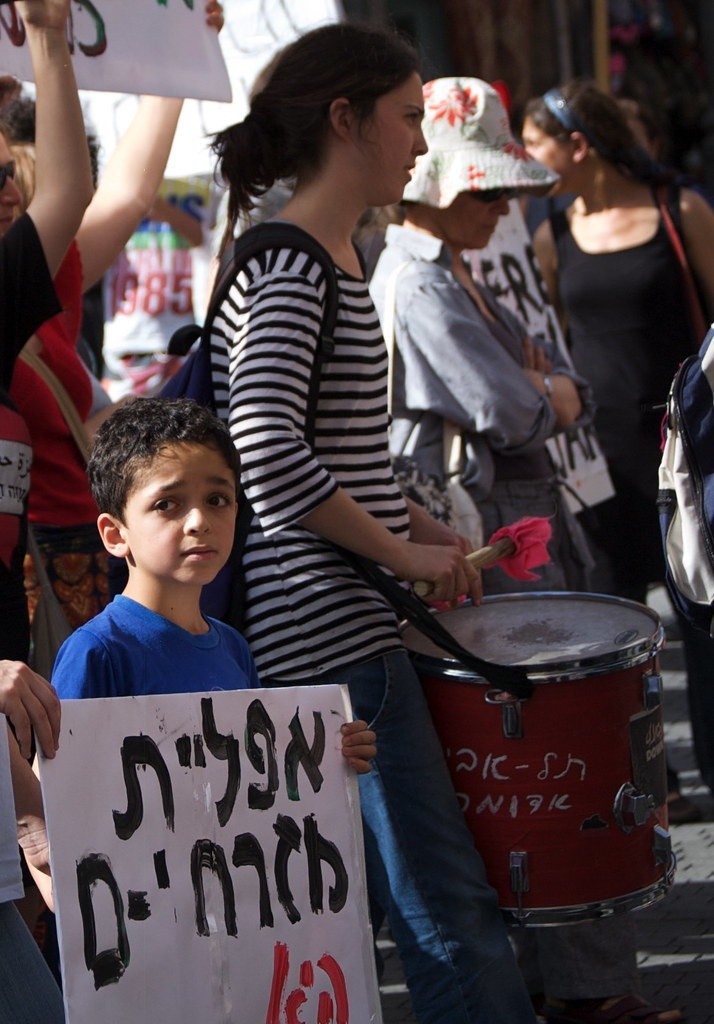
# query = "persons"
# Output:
<box><xmin>199</xmin><ymin>18</ymin><xmax>546</xmax><ymax>1024</ymax></box>
<box><xmin>360</xmin><ymin>71</ymin><xmax>714</xmax><ymax>1019</ymax></box>
<box><xmin>34</xmin><ymin>399</ymin><xmax>376</xmax><ymax>1024</ymax></box>
<box><xmin>0</xmin><ymin>0</ymin><xmax>218</xmax><ymax>1024</ymax></box>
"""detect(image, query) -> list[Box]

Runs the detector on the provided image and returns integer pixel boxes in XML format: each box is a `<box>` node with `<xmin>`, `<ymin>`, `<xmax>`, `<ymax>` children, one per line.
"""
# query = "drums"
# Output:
<box><xmin>394</xmin><ymin>589</ymin><xmax>678</xmax><ymax>930</ymax></box>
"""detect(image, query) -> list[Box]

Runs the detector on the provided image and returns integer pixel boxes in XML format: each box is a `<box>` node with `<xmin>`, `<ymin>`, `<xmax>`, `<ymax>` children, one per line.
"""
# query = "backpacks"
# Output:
<box><xmin>157</xmin><ymin>221</ymin><xmax>339</xmax><ymax>461</ymax></box>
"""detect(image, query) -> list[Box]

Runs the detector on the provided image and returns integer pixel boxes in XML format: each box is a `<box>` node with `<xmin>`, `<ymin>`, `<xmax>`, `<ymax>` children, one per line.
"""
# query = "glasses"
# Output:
<box><xmin>467</xmin><ymin>188</ymin><xmax>519</xmax><ymax>202</ymax></box>
<box><xmin>0</xmin><ymin>160</ymin><xmax>16</xmax><ymax>193</ymax></box>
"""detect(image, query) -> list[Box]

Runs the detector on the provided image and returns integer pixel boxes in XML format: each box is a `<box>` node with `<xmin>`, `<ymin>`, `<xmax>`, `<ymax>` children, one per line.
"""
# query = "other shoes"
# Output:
<box><xmin>598</xmin><ymin>993</ymin><xmax>682</xmax><ymax>1024</ymax></box>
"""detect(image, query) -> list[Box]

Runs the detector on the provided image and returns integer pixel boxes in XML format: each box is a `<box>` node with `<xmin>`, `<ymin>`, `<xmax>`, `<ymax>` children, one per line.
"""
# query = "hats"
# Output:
<box><xmin>396</xmin><ymin>76</ymin><xmax>561</xmax><ymax>209</ymax></box>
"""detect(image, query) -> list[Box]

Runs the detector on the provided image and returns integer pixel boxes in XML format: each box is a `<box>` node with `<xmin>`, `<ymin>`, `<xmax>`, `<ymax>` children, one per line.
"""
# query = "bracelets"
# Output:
<box><xmin>542</xmin><ymin>376</ymin><xmax>554</xmax><ymax>398</ymax></box>
